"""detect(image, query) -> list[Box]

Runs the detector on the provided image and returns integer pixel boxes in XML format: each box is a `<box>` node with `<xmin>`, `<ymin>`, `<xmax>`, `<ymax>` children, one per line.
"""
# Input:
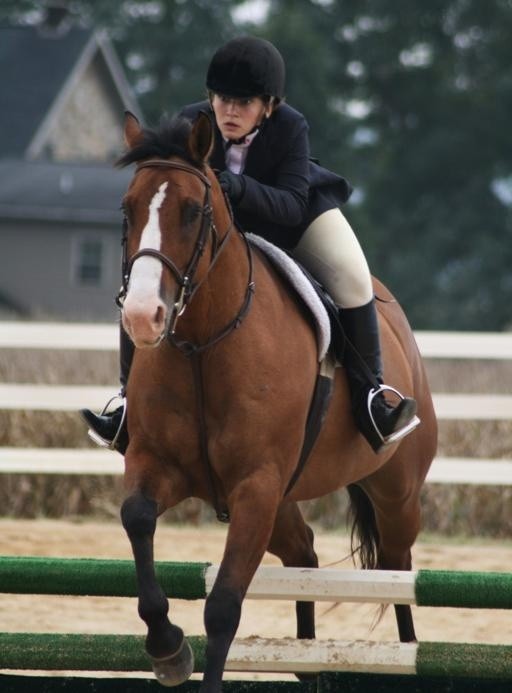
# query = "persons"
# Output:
<box><xmin>78</xmin><ymin>35</ymin><xmax>418</xmax><ymax>457</ymax></box>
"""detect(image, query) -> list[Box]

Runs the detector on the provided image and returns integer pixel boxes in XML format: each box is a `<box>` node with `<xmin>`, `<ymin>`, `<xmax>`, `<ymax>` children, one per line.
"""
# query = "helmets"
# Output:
<box><xmin>206</xmin><ymin>35</ymin><xmax>285</xmax><ymax>103</ymax></box>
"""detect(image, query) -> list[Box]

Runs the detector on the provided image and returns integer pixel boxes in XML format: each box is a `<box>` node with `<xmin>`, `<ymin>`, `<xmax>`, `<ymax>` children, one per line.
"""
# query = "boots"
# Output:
<box><xmin>79</xmin><ymin>307</ymin><xmax>135</xmax><ymax>455</ymax></box>
<box><xmin>333</xmin><ymin>297</ymin><xmax>417</xmax><ymax>455</ymax></box>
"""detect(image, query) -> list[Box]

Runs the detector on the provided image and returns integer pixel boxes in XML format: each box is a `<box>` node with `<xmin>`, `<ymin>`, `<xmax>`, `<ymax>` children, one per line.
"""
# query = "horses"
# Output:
<box><xmin>115</xmin><ymin>111</ymin><xmax>439</xmax><ymax>690</ymax></box>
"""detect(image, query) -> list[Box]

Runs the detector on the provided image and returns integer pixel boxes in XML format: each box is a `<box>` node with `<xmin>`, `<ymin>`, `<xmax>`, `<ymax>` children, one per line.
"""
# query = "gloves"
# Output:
<box><xmin>217</xmin><ymin>170</ymin><xmax>245</xmax><ymax>205</ymax></box>
